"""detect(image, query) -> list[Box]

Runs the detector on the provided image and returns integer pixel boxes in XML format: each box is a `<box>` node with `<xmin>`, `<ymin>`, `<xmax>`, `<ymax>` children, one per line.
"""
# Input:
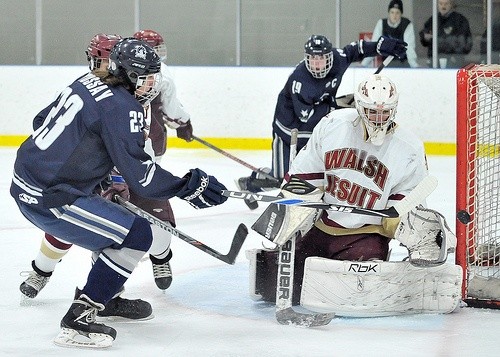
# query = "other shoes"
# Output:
<box><xmin>234</xmin><ymin>176</ymin><xmax>258</xmax><ymax>210</ymax></box>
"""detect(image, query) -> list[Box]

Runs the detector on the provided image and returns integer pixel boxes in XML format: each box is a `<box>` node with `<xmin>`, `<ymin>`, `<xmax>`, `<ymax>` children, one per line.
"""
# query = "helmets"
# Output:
<box><xmin>87</xmin><ymin>33</ymin><xmax>123</xmax><ymax>60</ymax></box>
<box><xmin>134</xmin><ymin>29</ymin><xmax>163</xmax><ymax>47</ymax></box>
<box><xmin>354</xmin><ymin>74</ymin><xmax>399</xmax><ymax>147</ymax></box>
<box><xmin>109</xmin><ymin>37</ymin><xmax>161</xmax><ymax>83</ymax></box>
<box><xmin>304</xmin><ymin>35</ymin><xmax>332</xmax><ymax>56</ymax></box>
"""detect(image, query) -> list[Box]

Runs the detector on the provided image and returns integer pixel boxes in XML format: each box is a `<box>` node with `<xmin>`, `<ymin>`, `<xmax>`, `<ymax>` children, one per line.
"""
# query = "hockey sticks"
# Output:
<box><xmin>163</xmin><ymin>119</ymin><xmax>280</xmax><ymax>182</ymax></box>
<box><xmin>347</xmin><ymin>55</ymin><xmax>395</xmax><ymax>106</ymax></box>
<box><xmin>221</xmin><ymin>173</ymin><xmax>438</xmax><ymax>218</ymax></box>
<box><xmin>275</xmin><ymin>128</ymin><xmax>336</xmax><ymax>327</ymax></box>
<box><xmin>111</xmin><ymin>193</ymin><xmax>248</xmax><ymax>265</ymax></box>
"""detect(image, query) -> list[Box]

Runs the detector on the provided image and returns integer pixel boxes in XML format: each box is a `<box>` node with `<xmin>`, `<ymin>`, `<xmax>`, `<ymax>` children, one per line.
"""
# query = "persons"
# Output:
<box><xmin>250</xmin><ymin>76</ymin><xmax>465</xmax><ymax>317</ymax></box>
<box><xmin>357</xmin><ymin>0</ymin><xmax>500</xmax><ymax>67</ymax></box>
<box><xmin>9</xmin><ymin>30</ymin><xmax>228</xmax><ymax>348</ymax></box>
<box><xmin>235</xmin><ymin>34</ymin><xmax>407</xmax><ymax>209</ymax></box>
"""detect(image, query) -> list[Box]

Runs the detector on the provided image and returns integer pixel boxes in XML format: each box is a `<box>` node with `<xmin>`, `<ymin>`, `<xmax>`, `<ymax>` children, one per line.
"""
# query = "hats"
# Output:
<box><xmin>388</xmin><ymin>0</ymin><xmax>403</xmax><ymax>14</ymax></box>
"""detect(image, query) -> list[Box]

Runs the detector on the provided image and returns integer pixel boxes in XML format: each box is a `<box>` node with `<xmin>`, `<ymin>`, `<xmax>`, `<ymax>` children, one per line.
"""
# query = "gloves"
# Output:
<box><xmin>376</xmin><ymin>36</ymin><xmax>408</xmax><ymax>60</ymax></box>
<box><xmin>177</xmin><ymin>168</ymin><xmax>229</xmax><ymax>210</ymax></box>
<box><xmin>176</xmin><ymin>120</ymin><xmax>193</xmax><ymax>142</ymax></box>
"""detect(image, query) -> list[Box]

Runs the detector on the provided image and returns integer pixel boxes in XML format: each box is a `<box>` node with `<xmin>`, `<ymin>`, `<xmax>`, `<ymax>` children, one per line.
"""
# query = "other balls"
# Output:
<box><xmin>457</xmin><ymin>210</ymin><xmax>471</xmax><ymax>224</ymax></box>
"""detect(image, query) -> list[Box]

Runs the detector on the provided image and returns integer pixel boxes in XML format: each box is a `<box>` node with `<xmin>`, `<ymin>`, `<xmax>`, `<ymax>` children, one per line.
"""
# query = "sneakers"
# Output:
<box><xmin>95</xmin><ymin>296</ymin><xmax>156</xmax><ymax>324</ymax></box>
<box><xmin>53</xmin><ymin>287</ymin><xmax>117</xmax><ymax>348</ymax></box>
<box><xmin>149</xmin><ymin>249</ymin><xmax>173</xmax><ymax>296</ymax></box>
<box><xmin>19</xmin><ymin>260</ymin><xmax>54</xmax><ymax>306</ymax></box>
